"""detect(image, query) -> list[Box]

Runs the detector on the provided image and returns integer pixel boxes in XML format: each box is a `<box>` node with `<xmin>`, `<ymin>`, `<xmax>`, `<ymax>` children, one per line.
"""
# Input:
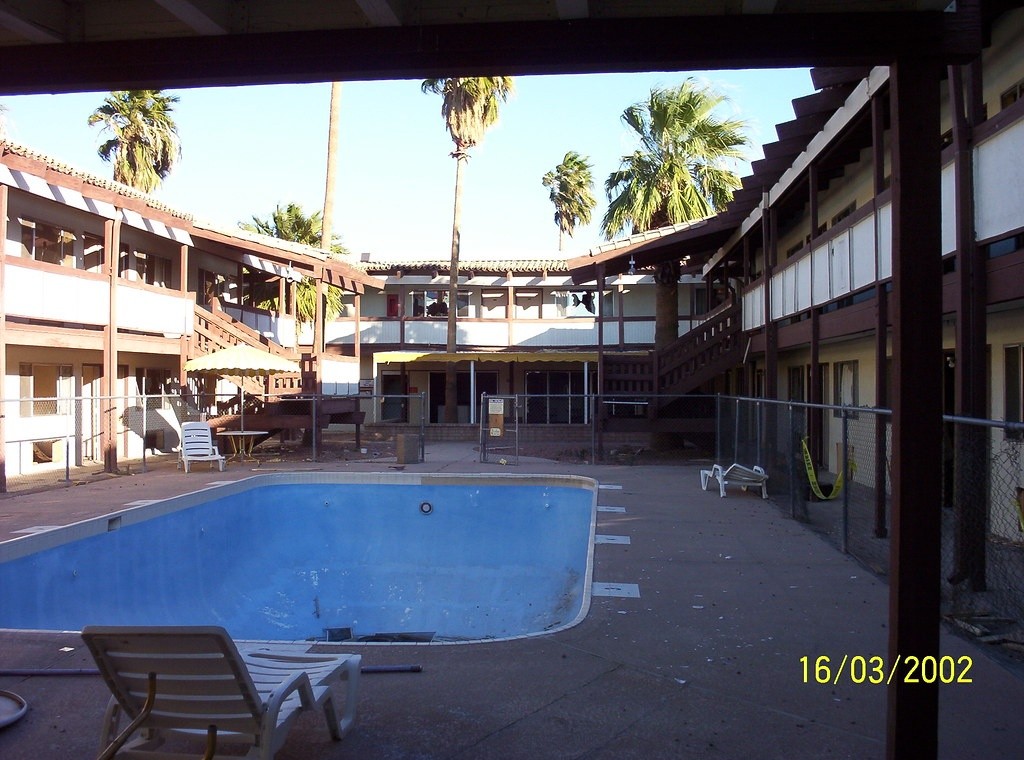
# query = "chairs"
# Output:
<box><xmin>173</xmin><ymin>423</ymin><xmax>226</xmax><ymax>472</ymax></box>
<box><xmin>700</xmin><ymin>464</ymin><xmax>768</xmax><ymax>499</ymax></box>
<box><xmin>79</xmin><ymin>624</ymin><xmax>362</xmax><ymax>760</ymax></box>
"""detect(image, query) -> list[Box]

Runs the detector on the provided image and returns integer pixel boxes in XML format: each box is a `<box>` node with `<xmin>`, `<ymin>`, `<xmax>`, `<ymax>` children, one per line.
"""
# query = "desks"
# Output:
<box><xmin>218</xmin><ymin>432</ymin><xmax>267</xmax><ymax>466</ymax></box>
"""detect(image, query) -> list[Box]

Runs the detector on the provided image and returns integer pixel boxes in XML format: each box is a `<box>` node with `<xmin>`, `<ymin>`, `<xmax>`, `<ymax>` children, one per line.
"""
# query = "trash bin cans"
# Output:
<box><xmin>634</xmin><ymin>399</ymin><xmax>647</xmax><ymax>417</ymax></box>
<box><xmin>396</xmin><ymin>433</ymin><xmax>420</xmax><ymax>464</ymax></box>
